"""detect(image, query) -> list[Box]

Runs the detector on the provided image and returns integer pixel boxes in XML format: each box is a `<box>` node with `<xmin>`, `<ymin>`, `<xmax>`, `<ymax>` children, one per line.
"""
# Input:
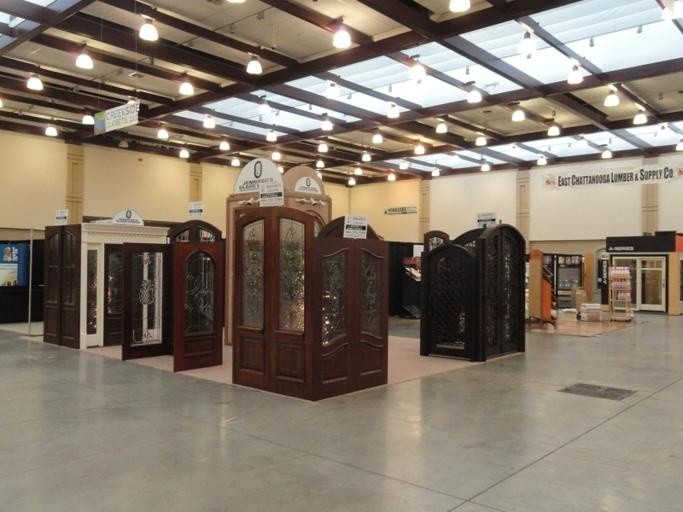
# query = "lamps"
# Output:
<box><xmin>22</xmin><ymin>0</ymin><xmax>682</xmax><ymax>185</ymax></box>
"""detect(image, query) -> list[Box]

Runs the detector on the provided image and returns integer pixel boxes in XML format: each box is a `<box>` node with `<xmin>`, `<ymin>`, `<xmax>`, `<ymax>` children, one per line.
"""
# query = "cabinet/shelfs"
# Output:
<box><xmin>608</xmin><ymin>267</ymin><xmax>634</xmax><ymax>322</ymax></box>
<box><xmin>528</xmin><ymin>249</ymin><xmax>558</xmax><ymax>330</ymax></box>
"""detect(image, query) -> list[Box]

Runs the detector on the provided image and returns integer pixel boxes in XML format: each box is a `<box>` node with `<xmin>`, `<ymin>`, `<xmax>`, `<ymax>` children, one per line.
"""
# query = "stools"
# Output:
<box><xmin>580</xmin><ymin>302</ymin><xmax>602</xmax><ymax>322</ymax></box>
